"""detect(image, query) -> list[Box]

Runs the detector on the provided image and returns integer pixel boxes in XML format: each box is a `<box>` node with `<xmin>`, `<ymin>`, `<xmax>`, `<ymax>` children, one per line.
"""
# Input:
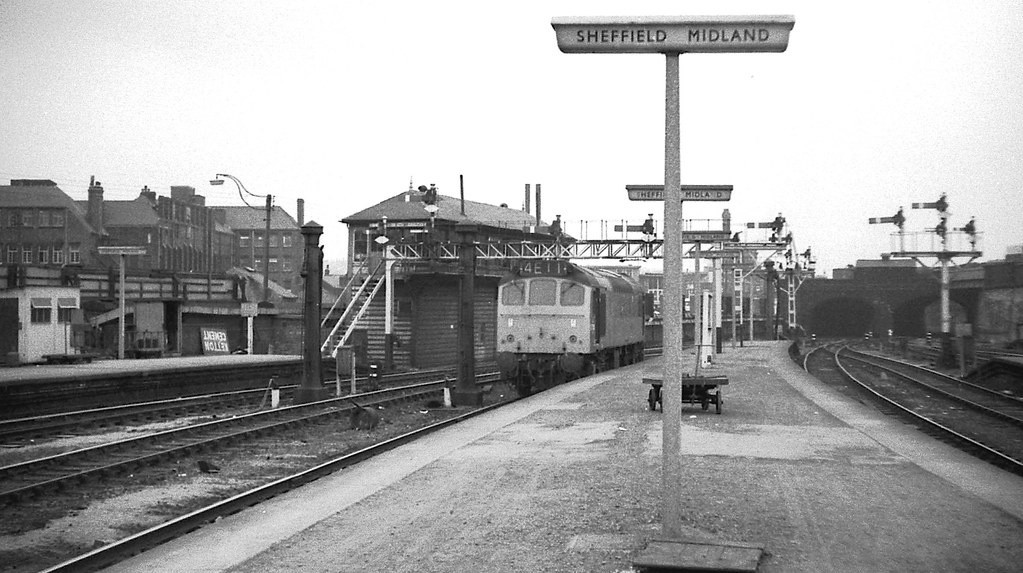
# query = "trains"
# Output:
<box><xmin>496</xmin><ymin>259</ymin><xmax>645</xmax><ymax>398</ymax></box>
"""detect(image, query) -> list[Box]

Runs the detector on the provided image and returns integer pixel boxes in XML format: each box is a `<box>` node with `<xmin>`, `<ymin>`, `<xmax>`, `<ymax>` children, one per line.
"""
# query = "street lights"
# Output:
<box><xmin>210</xmin><ymin>174</ymin><xmax>271</xmax><ymax>301</ymax></box>
